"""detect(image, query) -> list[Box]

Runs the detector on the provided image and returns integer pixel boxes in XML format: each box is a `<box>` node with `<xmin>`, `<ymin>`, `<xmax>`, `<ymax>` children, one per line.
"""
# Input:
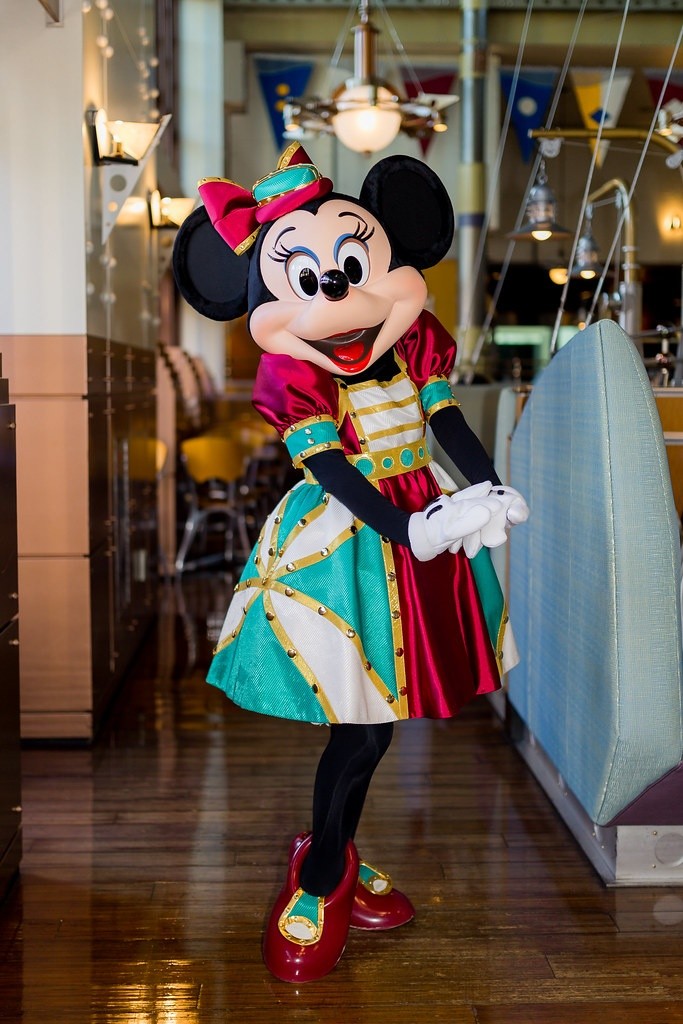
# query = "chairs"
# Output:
<box><xmin>174</xmin><ymin>413</ymin><xmax>285</xmax><ymax>588</ymax></box>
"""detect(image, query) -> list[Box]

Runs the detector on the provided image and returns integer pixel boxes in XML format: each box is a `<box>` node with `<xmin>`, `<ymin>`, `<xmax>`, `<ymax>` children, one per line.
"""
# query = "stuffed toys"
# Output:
<box><xmin>167</xmin><ymin>142</ymin><xmax>530</xmax><ymax>984</ymax></box>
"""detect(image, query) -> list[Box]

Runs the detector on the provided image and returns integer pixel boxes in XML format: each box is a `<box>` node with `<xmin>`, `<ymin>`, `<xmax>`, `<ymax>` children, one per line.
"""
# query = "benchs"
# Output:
<box><xmin>506</xmin><ymin>317</ymin><xmax>683</xmax><ymax>891</ymax></box>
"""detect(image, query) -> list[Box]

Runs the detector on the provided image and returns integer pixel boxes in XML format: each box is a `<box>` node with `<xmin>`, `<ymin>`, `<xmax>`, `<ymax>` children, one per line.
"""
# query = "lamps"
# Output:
<box><xmin>505</xmin><ymin>99</ymin><xmax>683</xmax><ymax>285</ymax></box>
<box><xmin>284</xmin><ymin>0</ymin><xmax>460</xmax><ymax>154</ymax></box>
<box><xmin>86</xmin><ymin>106</ymin><xmax>173</xmax><ymax>246</ymax></box>
<box><xmin>151</xmin><ymin>190</ymin><xmax>196</xmax><ymax>229</ymax></box>
